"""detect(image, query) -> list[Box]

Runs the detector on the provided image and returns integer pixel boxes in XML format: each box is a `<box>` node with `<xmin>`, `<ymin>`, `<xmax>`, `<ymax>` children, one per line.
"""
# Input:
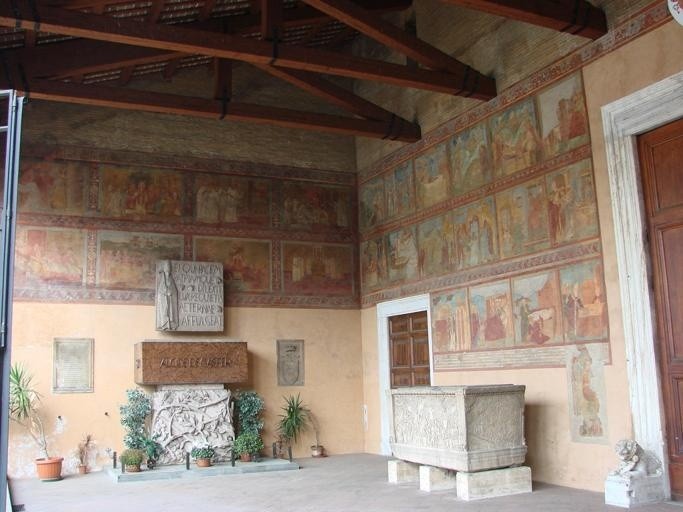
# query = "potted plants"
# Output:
<box><xmin>9</xmin><ymin>364</ymin><xmax>67</xmax><ymax>480</ymax></box>
<box><xmin>190</xmin><ymin>446</ymin><xmax>215</xmax><ymax>467</ymax></box>
<box><xmin>272</xmin><ymin>390</ymin><xmax>324</xmax><ymax>457</ymax></box>
<box><xmin>118</xmin><ymin>449</ymin><xmax>143</xmax><ymax>472</ymax></box>
<box><xmin>229</xmin><ymin>431</ymin><xmax>264</xmax><ymax>463</ymax></box>
<box><xmin>71</xmin><ymin>432</ymin><xmax>92</xmax><ymax>475</ymax></box>
